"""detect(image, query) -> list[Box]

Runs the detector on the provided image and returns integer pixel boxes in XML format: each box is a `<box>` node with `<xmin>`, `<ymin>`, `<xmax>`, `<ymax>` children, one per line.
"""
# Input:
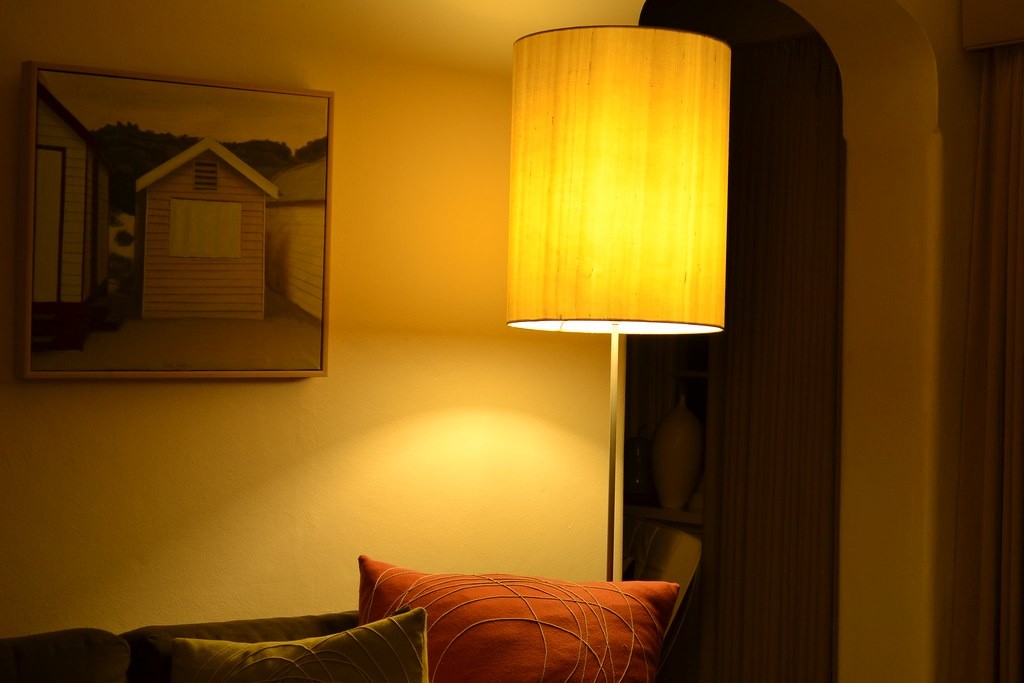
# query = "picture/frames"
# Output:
<box><xmin>15</xmin><ymin>57</ymin><xmax>336</xmax><ymax>378</ymax></box>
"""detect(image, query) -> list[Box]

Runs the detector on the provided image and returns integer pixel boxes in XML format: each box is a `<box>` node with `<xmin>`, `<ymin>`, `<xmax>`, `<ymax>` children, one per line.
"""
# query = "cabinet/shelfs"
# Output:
<box><xmin>624</xmin><ymin>333</ymin><xmax>723</xmax><ymax>683</ymax></box>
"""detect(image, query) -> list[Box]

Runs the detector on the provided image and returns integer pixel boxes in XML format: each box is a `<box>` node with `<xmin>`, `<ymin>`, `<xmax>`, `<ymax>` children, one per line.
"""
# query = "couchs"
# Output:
<box><xmin>0</xmin><ymin>609</ymin><xmax>361</xmax><ymax>683</ymax></box>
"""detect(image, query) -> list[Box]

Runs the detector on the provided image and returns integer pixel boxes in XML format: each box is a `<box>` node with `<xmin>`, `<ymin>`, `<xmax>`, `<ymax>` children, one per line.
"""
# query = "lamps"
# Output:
<box><xmin>504</xmin><ymin>24</ymin><xmax>734</xmax><ymax>577</ymax></box>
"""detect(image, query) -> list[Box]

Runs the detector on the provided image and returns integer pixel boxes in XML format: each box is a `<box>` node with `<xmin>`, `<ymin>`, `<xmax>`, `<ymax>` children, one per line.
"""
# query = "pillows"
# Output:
<box><xmin>359</xmin><ymin>554</ymin><xmax>679</xmax><ymax>683</ymax></box>
<box><xmin>167</xmin><ymin>611</ymin><xmax>430</xmax><ymax>683</ymax></box>
